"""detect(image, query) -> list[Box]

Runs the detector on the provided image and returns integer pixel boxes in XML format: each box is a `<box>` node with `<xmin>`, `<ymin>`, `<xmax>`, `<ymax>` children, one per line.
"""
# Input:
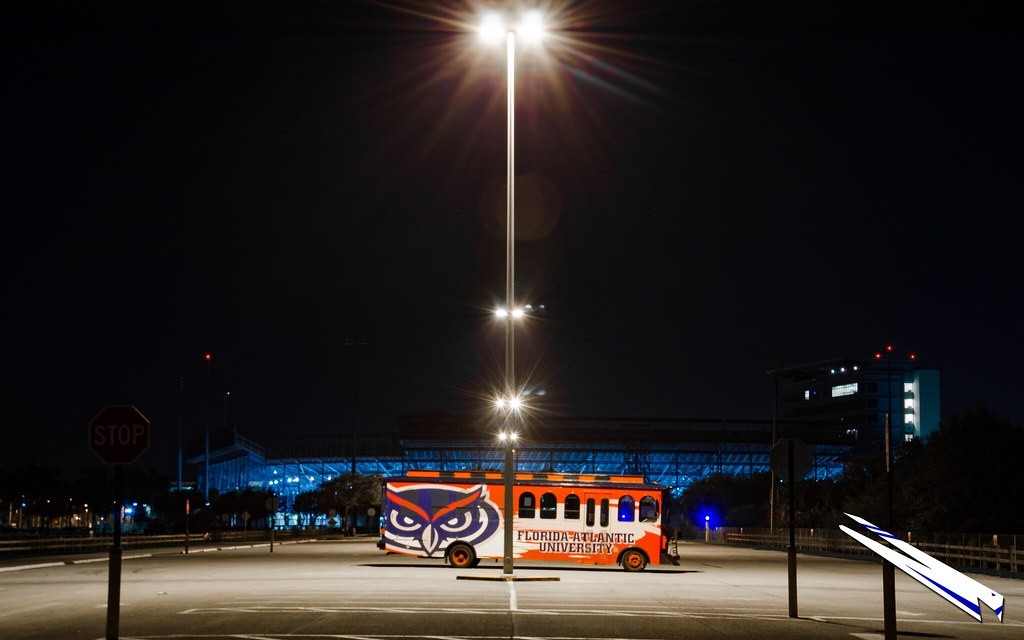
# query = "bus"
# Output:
<box><xmin>377</xmin><ymin>472</ymin><xmax>681</xmax><ymax>573</ymax></box>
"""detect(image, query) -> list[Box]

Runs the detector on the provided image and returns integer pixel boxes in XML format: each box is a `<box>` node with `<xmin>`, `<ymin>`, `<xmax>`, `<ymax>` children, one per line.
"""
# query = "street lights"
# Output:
<box><xmin>18</xmin><ymin>502</ymin><xmax>26</xmax><ymax>532</ymax></box>
<box><xmin>479</xmin><ymin>10</ymin><xmax>549</xmax><ymax>574</ymax></box>
